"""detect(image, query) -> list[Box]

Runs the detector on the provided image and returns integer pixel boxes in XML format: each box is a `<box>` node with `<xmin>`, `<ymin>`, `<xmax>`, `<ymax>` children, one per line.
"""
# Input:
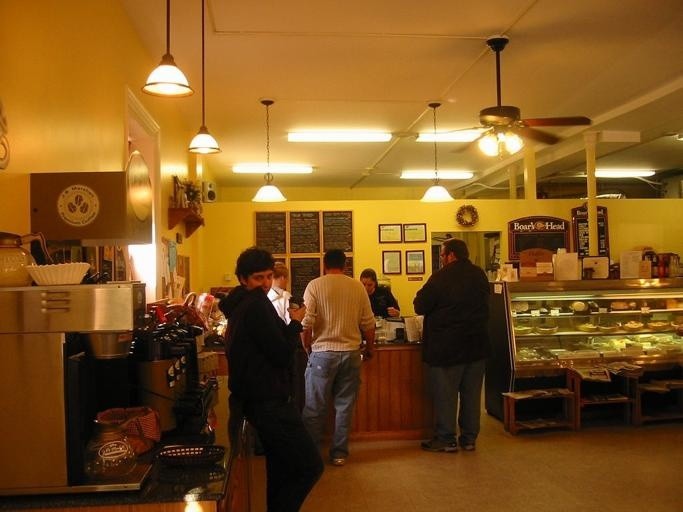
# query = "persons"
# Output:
<box><xmin>218</xmin><ymin>246</ymin><xmax>325</xmax><ymax>512</ymax></box>
<box><xmin>302</xmin><ymin>249</ymin><xmax>376</xmax><ymax>466</ymax></box>
<box><xmin>361</xmin><ymin>268</ymin><xmax>398</xmax><ymax>318</ymax></box>
<box><xmin>265</xmin><ymin>261</ymin><xmax>293</xmax><ymax>329</ymax></box>
<box><xmin>414</xmin><ymin>238</ymin><xmax>492</xmax><ymax>453</ymax></box>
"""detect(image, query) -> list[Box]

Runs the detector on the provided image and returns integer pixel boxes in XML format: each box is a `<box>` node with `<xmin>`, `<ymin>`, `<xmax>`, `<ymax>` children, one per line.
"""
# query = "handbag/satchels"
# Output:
<box><xmin>169</xmin><ymin>293</ymin><xmax>209</xmax><ymax>334</ymax></box>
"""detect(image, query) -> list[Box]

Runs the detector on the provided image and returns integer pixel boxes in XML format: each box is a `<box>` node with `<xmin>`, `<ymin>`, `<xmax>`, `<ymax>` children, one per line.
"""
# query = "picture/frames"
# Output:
<box><xmin>404</xmin><ymin>223</ymin><xmax>427</xmax><ymax>243</ymax></box>
<box><xmin>378</xmin><ymin>223</ymin><xmax>402</xmax><ymax>243</ymax></box>
<box><xmin>382</xmin><ymin>250</ymin><xmax>402</xmax><ymax>274</ymax></box>
<box><xmin>406</xmin><ymin>250</ymin><xmax>425</xmax><ymax>274</ymax></box>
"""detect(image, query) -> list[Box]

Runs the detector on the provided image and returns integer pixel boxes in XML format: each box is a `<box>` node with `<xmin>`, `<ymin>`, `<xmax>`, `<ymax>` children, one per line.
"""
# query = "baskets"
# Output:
<box><xmin>155</xmin><ymin>444</ymin><xmax>226</xmax><ymax>467</ymax></box>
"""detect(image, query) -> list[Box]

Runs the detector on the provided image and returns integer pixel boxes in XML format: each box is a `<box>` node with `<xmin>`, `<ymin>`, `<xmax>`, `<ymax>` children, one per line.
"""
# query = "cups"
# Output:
<box><xmin>288</xmin><ymin>297</ymin><xmax>305</xmax><ymax>319</ymax></box>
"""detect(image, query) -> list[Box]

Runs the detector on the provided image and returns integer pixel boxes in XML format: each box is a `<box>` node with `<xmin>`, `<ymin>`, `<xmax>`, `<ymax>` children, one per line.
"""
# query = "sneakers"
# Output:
<box><xmin>459</xmin><ymin>435</ymin><xmax>477</xmax><ymax>453</ymax></box>
<box><xmin>332</xmin><ymin>455</ymin><xmax>345</xmax><ymax>467</ymax></box>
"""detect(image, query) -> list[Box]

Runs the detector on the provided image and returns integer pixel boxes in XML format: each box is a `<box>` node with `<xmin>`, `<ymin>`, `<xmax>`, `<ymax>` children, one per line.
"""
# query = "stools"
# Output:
<box><xmin>501</xmin><ymin>388</ymin><xmax>576</xmax><ymax>436</ymax></box>
<box><xmin>638</xmin><ymin>381</ymin><xmax>667</xmax><ymax>424</ymax></box>
<box><xmin>563</xmin><ymin>361</ymin><xmax>640</xmax><ymax>426</ymax></box>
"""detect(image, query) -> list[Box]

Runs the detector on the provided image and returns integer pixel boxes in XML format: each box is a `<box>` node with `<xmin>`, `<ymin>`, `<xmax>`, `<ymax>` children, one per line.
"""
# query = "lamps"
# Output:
<box><xmin>478</xmin><ymin>130</ymin><xmax>522</xmax><ymax>157</ymax></box>
<box><xmin>142</xmin><ymin>1</ymin><xmax>193</xmax><ymax>99</ymax></box>
<box><xmin>288</xmin><ymin>131</ymin><xmax>390</xmax><ymax>143</ymax></box>
<box><xmin>595</xmin><ymin>169</ymin><xmax>656</xmax><ymax>178</ymax></box>
<box><xmin>400</xmin><ymin>170</ymin><xmax>471</xmax><ymax>180</ymax></box>
<box><xmin>420</xmin><ymin>103</ymin><xmax>451</xmax><ymax>203</ymax></box>
<box><xmin>188</xmin><ymin>0</ymin><xmax>220</xmax><ymax>155</ymax></box>
<box><xmin>232</xmin><ymin>163</ymin><xmax>314</xmax><ymax>176</ymax></box>
<box><xmin>417</xmin><ymin>129</ymin><xmax>482</xmax><ymax>142</ymax></box>
<box><xmin>252</xmin><ymin>100</ymin><xmax>285</xmax><ymax>201</ymax></box>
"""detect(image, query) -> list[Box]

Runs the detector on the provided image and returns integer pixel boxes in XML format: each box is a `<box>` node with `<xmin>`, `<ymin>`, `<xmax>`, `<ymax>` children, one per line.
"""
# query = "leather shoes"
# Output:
<box><xmin>420</xmin><ymin>434</ymin><xmax>456</xmax><ymax>453</ymax></box>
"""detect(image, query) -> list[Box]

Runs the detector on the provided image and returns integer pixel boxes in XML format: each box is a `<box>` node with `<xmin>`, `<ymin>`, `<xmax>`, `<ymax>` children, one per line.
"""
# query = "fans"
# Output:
<box><xmin>434</xmin><ymin>39</ymin><xmax>591</xmax><ymax>153</ymax></box>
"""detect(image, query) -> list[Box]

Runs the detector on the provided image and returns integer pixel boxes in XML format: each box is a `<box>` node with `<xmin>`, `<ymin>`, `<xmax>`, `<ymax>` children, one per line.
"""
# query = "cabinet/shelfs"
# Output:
<box><xmin>486</xmin><ymin>278</ymin><xmax>683</xmax><ymax>420</ymax></box>
